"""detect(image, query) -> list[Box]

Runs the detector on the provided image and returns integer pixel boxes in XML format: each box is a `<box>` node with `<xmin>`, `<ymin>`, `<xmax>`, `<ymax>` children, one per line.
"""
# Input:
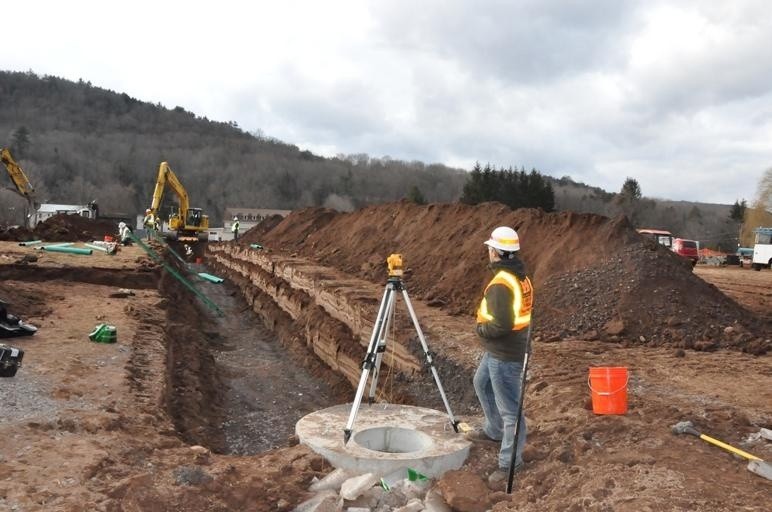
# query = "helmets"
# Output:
<box><xmin>483</xmin><ymin>226</ymin><xmax>521</xmax><ymax>252</ymax></box>
<box><xmin>146</xmin><ymin>209</ymin><xmax>151</xmax><ymax>213</ymax></box>
<box><xmin>119</xmin><ymin>222</ymin><xmax>126</xmax><ymax>228</ymax></box>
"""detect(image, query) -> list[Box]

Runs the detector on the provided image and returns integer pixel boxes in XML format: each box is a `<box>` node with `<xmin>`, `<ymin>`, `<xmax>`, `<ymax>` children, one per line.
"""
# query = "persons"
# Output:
<box><xmin>91</xmin><ymin>199</ymin><xmax>97</xmax><ymax>212</ymax></box>
<box><xmin>143</xmin><ymin>208</ymin><xmax>155</xmax><ymax>244</ymax></box>
<box><xmin>230</xmin><ymin>216</ymin><xmax>240</xmax><ymax>240</ymax></box>
<box><xmin>87</xmin><ymin>201</ymin><xmax>92</xmax><ymax>210</ymax></box>
<box><xmin>117</xmin><ymin>220</ymin><xmax>132</xmax><ymax>246</ymax></box>
<box><xmin>183</xmin><ymin>244</ymin><xmax>193</xmax><ymax>261</ymax></box>
<box><xmin>153</xmin><ymin>215</ymin><xmax>161</xmax><ymax>235</ymax></box>
<box><xmin>192</xmin><ymin>210</ymin><xmax>199</xmax><ymax>226</ymax></box>
<box><xmin>461</xmin><ymin>223</ymin><xmax>535</xmax><ymax>492</ymax></box>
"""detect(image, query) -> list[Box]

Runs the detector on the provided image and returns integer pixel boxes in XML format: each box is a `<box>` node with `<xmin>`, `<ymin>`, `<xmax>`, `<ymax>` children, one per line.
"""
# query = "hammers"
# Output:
<box><xmin>672</xmin><ymin>421</ymin><xmax>763</xmax><ymax>461</ymax></box>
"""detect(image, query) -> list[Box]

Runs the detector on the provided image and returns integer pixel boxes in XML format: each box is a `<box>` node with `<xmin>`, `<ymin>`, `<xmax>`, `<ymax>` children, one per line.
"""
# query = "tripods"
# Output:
<box><xmin>343</xmin><ymin>276</ymin><xmax>459</xmax><ymax>444</ymax></box>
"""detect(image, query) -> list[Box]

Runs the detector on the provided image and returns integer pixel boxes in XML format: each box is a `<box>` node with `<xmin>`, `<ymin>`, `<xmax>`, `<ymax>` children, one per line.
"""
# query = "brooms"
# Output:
<box><xmin>672</xmin><ymin>421</ymin><xmax>765</xmax><ymax>463</ymax></box>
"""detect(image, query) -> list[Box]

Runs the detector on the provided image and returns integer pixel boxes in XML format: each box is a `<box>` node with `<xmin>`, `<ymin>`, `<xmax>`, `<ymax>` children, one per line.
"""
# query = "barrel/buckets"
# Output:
<box><xmin>589</xmin><ymin>366</ymin><xmax>629</xmax><ymax>415</ymax></box>
<box><xmin>196</xmin><ymin>258</ymin><xmax>203</xmax><ymax>264</ymax></box>
<box><xmin>102</xmin><ymin>326</ymin><xmax>116</xmax><ymax>343</ymax></box>
<box><xmin>104</xmin><ymin>236</ymin><xmax>112</xmax><ymax>242</ymax></box>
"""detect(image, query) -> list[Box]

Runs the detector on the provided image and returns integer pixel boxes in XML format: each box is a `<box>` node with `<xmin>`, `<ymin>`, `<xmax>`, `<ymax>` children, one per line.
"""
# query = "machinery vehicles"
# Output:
<box><xmin>0</xmin><ymin>147</ymin><xmax>41</xmax><ymax>232</ymax></box>
<box><xmin>149</xmin><ymin>162</ymin><xmax>210</xmax><ymax>242</ymax></box>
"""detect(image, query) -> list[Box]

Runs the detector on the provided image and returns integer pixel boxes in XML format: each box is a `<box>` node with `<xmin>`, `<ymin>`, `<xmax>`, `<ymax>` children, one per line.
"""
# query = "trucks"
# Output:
<box><xmin>635</xmin><ymin>227</ymin><xmax>698</xmax><ymax>267</ymax></box>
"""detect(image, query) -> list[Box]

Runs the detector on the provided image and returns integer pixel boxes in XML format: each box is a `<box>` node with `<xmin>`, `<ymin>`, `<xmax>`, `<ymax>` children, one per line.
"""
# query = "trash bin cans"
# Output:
<box><xmin>209</xmin><ymin>232</ymin><xmax>218</xmax><ymax>242</ymax></box>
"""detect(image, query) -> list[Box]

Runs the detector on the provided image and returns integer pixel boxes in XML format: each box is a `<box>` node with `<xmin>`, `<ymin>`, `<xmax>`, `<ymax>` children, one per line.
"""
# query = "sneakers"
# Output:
<box><xmin>466</xmin><ymin>430</ymin><xmax>502</xmax><ymax>444</ymax></box>
<box><xmin>487</xmin><ymin>459</ymin><xmax>523</xmax><ymax>484</ymax></box>
